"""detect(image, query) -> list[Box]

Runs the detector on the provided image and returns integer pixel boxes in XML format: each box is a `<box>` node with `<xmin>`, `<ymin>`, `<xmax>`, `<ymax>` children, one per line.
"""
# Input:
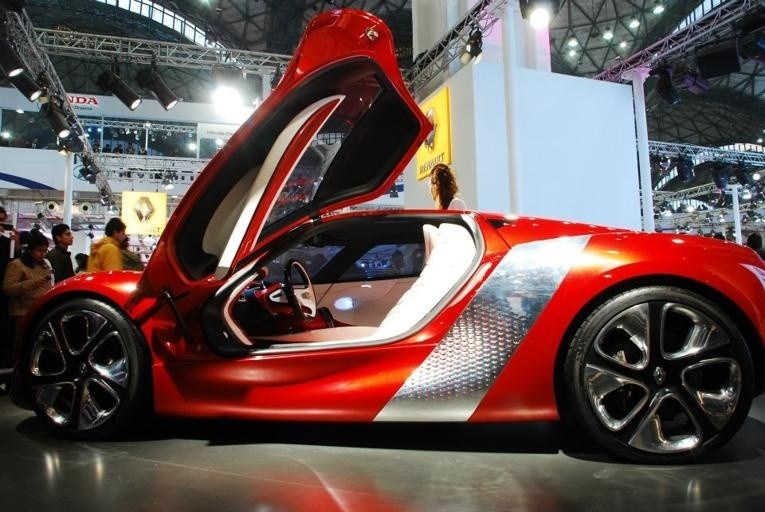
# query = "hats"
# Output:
<box><xmin>28</xmin><ymin>228</ymin><xmax>49</xmax><ymax>246</ymax></box>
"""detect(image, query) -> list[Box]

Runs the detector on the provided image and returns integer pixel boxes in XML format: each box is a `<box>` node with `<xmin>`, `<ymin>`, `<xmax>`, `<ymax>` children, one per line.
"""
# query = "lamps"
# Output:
<box><xmin>464</xmin><ymin>21</ymin><xmax>485</xmax><ymax>60</ymax></box>
<box><xmin>650</xmin><ymin>64</ymin><xmax>681</xmax><ymax>107</ymax></box>
<box><xmin>672</xmin><ymin>151</ymin><xmax>759</xmax><ymax>195</ymax></box>
<box><xmin>0</xmin><ymin>20</ymin><xmax>180</xmax><ymax>184</ymax></box>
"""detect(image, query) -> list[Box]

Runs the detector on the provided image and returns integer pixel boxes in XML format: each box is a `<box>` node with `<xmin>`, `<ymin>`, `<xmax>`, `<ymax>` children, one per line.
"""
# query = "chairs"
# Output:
<box><xmin>269</xmin><ymin>222</ymin><xmax>478</xmax><ymax>349</ymax></box>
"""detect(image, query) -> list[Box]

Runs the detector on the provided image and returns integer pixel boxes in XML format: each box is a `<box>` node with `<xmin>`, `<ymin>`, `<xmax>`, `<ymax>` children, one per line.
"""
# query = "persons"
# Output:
<box><xmin>134</xmin><ymin>195</ymin><xmax>156</xmax><ymax>223</ymax></box>
<box><xmin>310</xmin><ymin>254</ymin><xmax>330</xmax><ymax>273</ymax></box>
<box><xmin>0</xmin><ymin>206</ymin><xmax>145</xmax><ymax>395</ymax></box>
<box><xmin>1</xmin><ymin>134</ymin><xmax>184</xmax><ymax>155</ymax></box>
<box><xmin>383</xmin><ymin>249</ymin><xmax>406</xmax><ymax>276</ymax></box>
<box><xmin>429</xmin><ymin>164</ymin><xmax>468</xmax><ymax>212</ymax></box>
<box><xmin>653</xmin><ymin>178</ymin><xmax>764</xmax><ymax>259</ymax></box>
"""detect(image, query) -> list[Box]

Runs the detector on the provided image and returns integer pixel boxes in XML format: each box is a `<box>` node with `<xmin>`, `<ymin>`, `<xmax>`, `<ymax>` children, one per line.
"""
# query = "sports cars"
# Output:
<box><xmin>11</xmin><ymin>2</ymin><xmax>765</xmax><ymax>469</ymax></box>
